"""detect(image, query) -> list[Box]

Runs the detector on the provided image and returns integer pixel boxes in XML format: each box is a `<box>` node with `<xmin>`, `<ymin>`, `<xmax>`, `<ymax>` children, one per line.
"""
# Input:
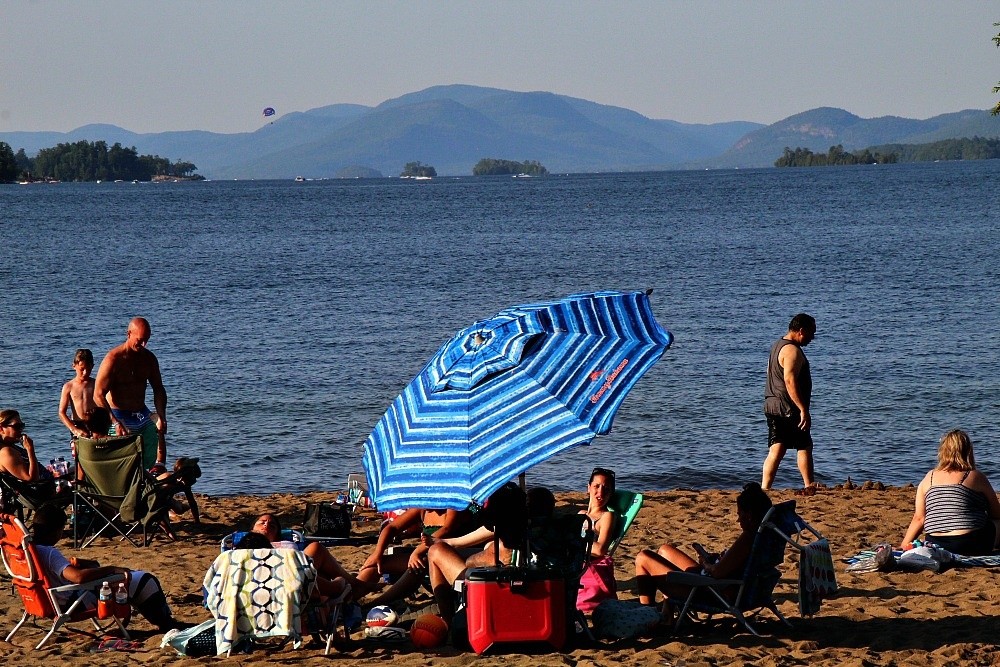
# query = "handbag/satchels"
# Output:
<box><xmin>901</xmin><ymin>544</ymin><xmax>961</xmax><ymax>573</ymax></box>
<box><xmin>303</xmin><ymin>500</ymin><xmax>351</xmax><ymax>539</ymax></box>
<box><xmin>845</xmin><ymin>541</ymin><xmax>897</xmax><ymax>574</ymax></box>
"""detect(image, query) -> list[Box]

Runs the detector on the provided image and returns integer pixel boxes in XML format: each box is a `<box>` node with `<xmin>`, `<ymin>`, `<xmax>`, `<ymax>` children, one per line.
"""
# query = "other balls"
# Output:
<box><xmin>410</xmin><ymin>614</ymin><xmax>448</xmax><ymax>654</ymax></box>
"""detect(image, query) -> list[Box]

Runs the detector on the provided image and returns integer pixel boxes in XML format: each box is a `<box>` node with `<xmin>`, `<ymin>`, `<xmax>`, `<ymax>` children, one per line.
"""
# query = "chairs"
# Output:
<box><xmin>0</xmin><ymin>513</ymin><xmax>131</xmax><ymax>650</ymax></box>
<box><xmin>74</xmin><ymin>433</ymin><xmax>176</xmax><ymax>548</ymax></box>
<box><xmin>666</xmin><ymin>500</ymin><xmax>824</xmax><ymax>637</ymax></box>
<box><xmin>574</xmin><ymin>490</ymin><xmax>644</xmax><ymax>643</ymax></box>
<box><xmin>220</xmin><ymin>530</ymin><xmax>305</xmax><ymax>551</ymax></box>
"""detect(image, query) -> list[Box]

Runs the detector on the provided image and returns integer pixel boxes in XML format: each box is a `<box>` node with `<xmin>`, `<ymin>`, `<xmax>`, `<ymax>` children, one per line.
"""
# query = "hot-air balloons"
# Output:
<box><xmin>262</xmin><ymin>107</ymin><xmax>276</xmax><ymax>125</ymax></box>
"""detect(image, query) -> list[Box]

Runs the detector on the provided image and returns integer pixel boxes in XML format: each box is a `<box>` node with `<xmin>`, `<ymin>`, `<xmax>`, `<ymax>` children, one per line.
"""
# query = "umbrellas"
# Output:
<box><xmin>363</xmin><ymin>285</ymin><xmax>676</xmax><ymax>511</ymax></box>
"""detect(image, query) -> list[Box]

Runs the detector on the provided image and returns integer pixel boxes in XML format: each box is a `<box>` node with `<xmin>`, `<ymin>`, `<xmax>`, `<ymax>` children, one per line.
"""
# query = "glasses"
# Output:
<box><xmin>592</xmin><ymin>468</ymin><xmax>615</xmax><ymax>479</ymax></box>
<box><xmin>6</xmin><ymin>423</ymin><xmax>25</xmax><ymax>431</ymax></box>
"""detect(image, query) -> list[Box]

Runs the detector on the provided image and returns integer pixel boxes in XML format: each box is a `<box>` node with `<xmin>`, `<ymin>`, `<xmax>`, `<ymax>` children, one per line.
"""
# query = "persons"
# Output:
<box><xmin>0</xmin><ymin>409</ymin><xmax>76</xmax><ymax>507</ymax></box>
<box><xmin>232</xmin><ymin>467</ymin><xmax>623</xmax><ymax>650</ymax></box>
<box><xmin>93</xmin><ymin>318</ymin><xmax>168</xmax><ymax>474</ymax></box>
<box><xmin>30</xmin><ymin>502</ymin><xmax>191</xmax><ymax>632</ymax></box>
<box><xmin>631</xmin><ymin>480</ymin><xmax>775</xmax><ymax>634</ymax></box>
<box><xmin>57</xmin><ymin>350</ymin><xmax>103</xmax><ymax>493</ymax></box>
<box><xmin>898</xmin><ymin>430</ymin><xmax>1000</xmax><ymax>559</ymax></box>
<box><xmin>147</xmin><ymin>456</ymin><xmax>202</xmax><ymax>540</ymax></box>
<box><xmin>76</xmin><ymin>406</ymin><xmax>136</xmax><ymax>492</ymax></box>
<box><xmin>761</xmin><ymin>314</ymin><xmax>819</xmax><ymax>489</ymax></box>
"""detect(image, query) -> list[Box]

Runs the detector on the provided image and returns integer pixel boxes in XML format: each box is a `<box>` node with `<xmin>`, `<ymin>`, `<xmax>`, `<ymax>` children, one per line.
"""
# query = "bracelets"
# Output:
<box><xmin>113</xmin><ymin>422</ymin><xmax>121</xmax><ymax>427</ymax></box>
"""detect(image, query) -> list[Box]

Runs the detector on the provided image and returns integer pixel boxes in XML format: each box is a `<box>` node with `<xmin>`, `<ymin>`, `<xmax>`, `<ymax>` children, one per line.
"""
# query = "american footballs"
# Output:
<box><xmin>366</xmin><ymin>605</ymin><xmax>399</xmax><ymax>628</ymax></box>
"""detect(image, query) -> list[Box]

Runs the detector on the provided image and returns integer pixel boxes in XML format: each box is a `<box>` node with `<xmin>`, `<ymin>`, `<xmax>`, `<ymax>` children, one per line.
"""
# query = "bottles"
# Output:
<box><xmin>100</xmin><ymin>582</ymin><xmax>112</xmax><ymax>600</ymax></box>
<box><xmin>115</xmin><ymin>582</ymin><xmax>128</xmax><ymax>604</ymax></box>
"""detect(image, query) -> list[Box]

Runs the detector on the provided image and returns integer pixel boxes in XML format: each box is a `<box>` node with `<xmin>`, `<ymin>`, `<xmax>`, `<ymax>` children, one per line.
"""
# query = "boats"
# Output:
<box><xmin>294</xmin><ymin>176</ymin><xmax>304</xmax><ymax>181</ymax></box>
<box><xmin>511</xmin><ymin>173</ymin><xmax>531</xmax><ymax>178</ymax></box>
<box><xmin>399</xmin><ymin>175</ymin><xmax>432</xmax><ymax>180</ymax></box>
<box><xmin>203</xmin><ymin>179</ymin><xmax>211</xmax><ymax>182</ymax></box>
<box><xmin>96</xmin><ymin>179</ymin><xmax>178</xmax><ymax>184</ymax></box>
<box><xmin>19</xmin><ymin>177</ymin><xmax>59</xmax><ymax>185</ymax></box>
